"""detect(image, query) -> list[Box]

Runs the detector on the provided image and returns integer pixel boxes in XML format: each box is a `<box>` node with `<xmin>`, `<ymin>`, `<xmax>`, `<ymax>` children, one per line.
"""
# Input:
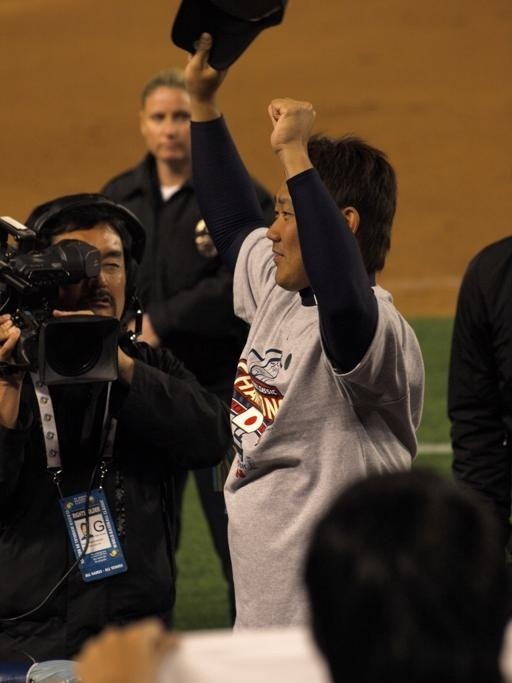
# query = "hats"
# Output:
<box><xmin>170</xmin><ymin>0</ymin><xmax>287</xmax><ymax>72</ymax></box>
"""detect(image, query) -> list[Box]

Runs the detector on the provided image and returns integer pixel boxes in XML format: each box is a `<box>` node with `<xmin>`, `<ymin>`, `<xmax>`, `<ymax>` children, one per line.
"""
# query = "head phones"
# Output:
<box><xmin>34</xmin><ymin>198</ymin><xmax>149</xmax><ymax>310</ymax></box>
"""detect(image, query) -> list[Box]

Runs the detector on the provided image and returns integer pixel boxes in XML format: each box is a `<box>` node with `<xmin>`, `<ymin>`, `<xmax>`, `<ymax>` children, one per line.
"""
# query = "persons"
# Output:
<box><xmin>3</xmin><ymin>186</ymin><xmax>231</xmax><ymax>671</ymax></box>
<box><xmin>68</xmin><ymin>466</ymin><xmax>510</xmax><ymax>682</ymax></box>
<box><xmin>173</xmin><ymin>30</ymin><xmax>427</xmax><ymax>632</ymax></box>
<box><xmin>446</xmin><ymin>234</ymin><xmax>510</xmax><ymax>503</ymax></box>
<box><xmin>87</xmin><ymin>64</ymin><xmax>236</xmax><ymax>632</ymax></box>
